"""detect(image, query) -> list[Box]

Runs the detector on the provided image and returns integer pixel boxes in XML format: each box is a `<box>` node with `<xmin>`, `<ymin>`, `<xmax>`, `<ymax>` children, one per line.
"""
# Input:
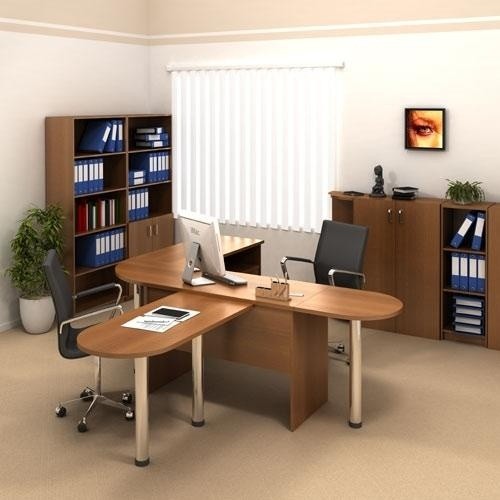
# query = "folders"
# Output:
<box><xmin>451</xmin><ymin>296</ymin><xmax>485</xmax><ymax>336</ymax></box>
<box><xmin>128</xmin><ymin>188</ymin><xmax>149</xmax><ymax>222</ymax></box>
<box><xmin>77</xmin><ymin>228</ymin><xmax>124</xmax><ymax>267</ymax></box>
<box><xmin>133</xmin><ymin>127</ymin><xmax>169</xmax><ymax>147</ymax></box>
<box><xmin>74</xmin><ymin>158</ymin><xmax>104</xmax><ymax>195</ymax></box>
<box><xmin>77</xmin><ymin>120</ymin><xmax>123</xmax><ymax>152</ymax></box>
<box><xmin>137</xmin><ymin>152</ymin><xmax>169</xmax><ymax>183</ymax></box>
<box><xmin>450</xmin><ymin>212</ymin><xmax>485</xmax><ymax>251</ymax></box>
<box><xmin>451</xmin><ymin>252</ymin><xmax>485</xmax><ymax>293</ymax></box>
<box><xmin>129</xmin><ymin>170</ymin><xmax>147</xmax><ymax>186</ymax></box>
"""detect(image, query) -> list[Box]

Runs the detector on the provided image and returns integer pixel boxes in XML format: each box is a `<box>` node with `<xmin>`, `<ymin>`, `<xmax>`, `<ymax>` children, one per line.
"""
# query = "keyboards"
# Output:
<box><xmin>202</xmin><ymin>273</ymin><xmax>248</xmax><ymax>286</ymax></box>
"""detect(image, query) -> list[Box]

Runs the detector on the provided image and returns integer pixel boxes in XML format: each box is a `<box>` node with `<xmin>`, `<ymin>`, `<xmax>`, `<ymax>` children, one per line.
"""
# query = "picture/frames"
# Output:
<box><xmin>404</xmin><ymin>108</ymin><xmax>446</xmax><ymax>150</ymax></box>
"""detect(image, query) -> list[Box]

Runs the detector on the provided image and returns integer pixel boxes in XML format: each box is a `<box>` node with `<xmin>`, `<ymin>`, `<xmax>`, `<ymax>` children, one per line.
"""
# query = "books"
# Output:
<box><xmin>391</xmin><ymin>186</ymin><xmax>420</xmax><ymax>200</ymax></box>
<box><xmin>448</xmin><ymin>211</ymin><xmax>485</xmax><ymax>335</ymax></box>
<box><xmin>73</xmin><ymin>117</ymin><xmax>172</xmax><ymax>270</ymax></box>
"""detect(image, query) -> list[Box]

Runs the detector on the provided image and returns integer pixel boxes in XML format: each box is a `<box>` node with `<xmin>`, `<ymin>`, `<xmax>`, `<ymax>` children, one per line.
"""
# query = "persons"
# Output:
<box><xmin>371</xmin><ymin>165</ymin><xmax>384</xmax><ymax>194</ymax></box>
<box><xmin>406</xmin><ymin>111</ymin><xmax>442</xmax><ymax>148</ymax></box>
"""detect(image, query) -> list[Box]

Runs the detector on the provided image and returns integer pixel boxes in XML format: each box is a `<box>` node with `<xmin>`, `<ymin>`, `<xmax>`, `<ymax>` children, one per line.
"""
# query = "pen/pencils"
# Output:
<box><xmin>143</xmin><ymin>320</ymin><xmax>169</xmax><ymax>323</ymax></box>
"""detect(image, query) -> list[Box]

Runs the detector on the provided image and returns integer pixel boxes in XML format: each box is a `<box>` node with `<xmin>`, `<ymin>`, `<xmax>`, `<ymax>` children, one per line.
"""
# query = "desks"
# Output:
<box><xmin>76</xmin><ymin>228</ymin><xmax>406</xmax><ymax>466</ymax></box>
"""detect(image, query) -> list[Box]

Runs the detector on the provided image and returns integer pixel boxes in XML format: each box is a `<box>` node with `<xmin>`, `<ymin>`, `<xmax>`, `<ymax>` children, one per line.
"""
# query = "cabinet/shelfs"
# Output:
<box><xmin>353</xmin><ymin>192</ymin><xmax>440</xmax><ymax>340</ymax></box>
<box><xmin>330</xmin><ymin>190</ymin><xmax>354</xmax><ymax>223</ymax></box>
<box><xmin>44</xmin><ymin>113</ymin><xmax>177</xmax><ymax>317</ymax></box>
<box><xmin>440</xmin><ymin>200</ymin><xmax>499</xmax><ymax>349</ymax></box>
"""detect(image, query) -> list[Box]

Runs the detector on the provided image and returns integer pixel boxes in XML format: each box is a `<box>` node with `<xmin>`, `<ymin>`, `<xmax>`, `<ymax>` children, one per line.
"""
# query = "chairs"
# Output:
<box><xmin>43</xmin><ymin>249</ymin><xmax>135</xmax><ymax>432</ymax></box>
<box><xmin>279</xmin><ymin>220</ymin><xmax>369</xmax><ymax>364</ymax></box>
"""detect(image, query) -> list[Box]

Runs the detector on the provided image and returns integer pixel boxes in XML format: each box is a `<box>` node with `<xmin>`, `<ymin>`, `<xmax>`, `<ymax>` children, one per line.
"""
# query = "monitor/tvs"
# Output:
<box><xmin>176</xmin><ymin>209</ymin><xmax>226</xmax><ymax>286</ymax></box>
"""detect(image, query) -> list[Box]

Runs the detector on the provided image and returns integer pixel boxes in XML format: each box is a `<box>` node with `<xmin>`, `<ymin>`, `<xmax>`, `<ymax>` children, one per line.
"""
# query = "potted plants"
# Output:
<box><xmin>3</xmin><ymin>201</ymin><xmax>71</xmax><ymax>335</ymax></box>
